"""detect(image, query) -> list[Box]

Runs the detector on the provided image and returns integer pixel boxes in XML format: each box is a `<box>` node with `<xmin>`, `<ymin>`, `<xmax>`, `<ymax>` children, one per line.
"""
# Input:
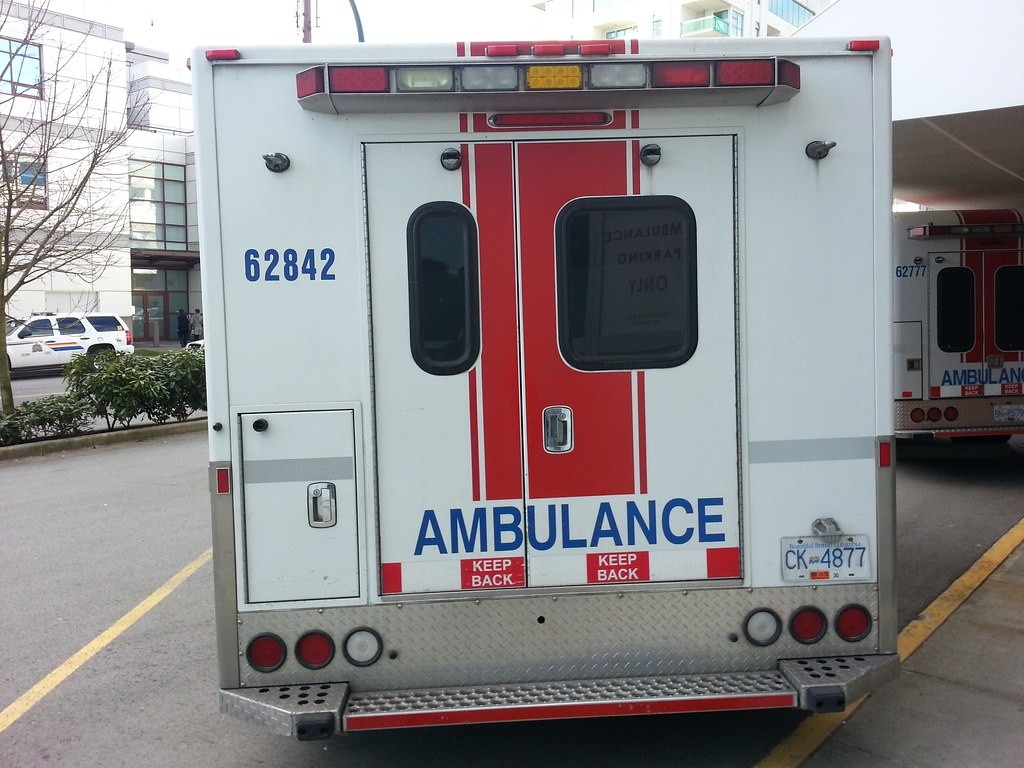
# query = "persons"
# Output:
<box><xmin>175</xmin><ymin>307</ymin><xmax>205</xmax><ymax>349</ymax></box>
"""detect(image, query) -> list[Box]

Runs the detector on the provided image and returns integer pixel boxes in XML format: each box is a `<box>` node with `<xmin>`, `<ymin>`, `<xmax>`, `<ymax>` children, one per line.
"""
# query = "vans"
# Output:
<box><xmin>5</xmin><ymin>312</ymin><xmax>135</xmax><ymax>379</ymax></box>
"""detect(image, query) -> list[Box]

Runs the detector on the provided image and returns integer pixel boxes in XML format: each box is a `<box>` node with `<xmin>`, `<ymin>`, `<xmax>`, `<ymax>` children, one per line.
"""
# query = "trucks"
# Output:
<box><xmin>190</xmin><ymin>35</ymin><xmax>898</xmax><ymax>741</ymax></box>
<box><xmin>892</xmin><ymin>207</ymin><xmax>1023</xmax><ymax>460</ymax></box>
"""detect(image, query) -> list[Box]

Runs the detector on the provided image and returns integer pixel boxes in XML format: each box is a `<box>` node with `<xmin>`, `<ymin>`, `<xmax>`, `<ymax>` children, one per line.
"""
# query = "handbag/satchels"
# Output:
<box><xmin>191</xmin><ymin>330</ymin><xmax>195</xmax><ymax>335</ymax></box>
